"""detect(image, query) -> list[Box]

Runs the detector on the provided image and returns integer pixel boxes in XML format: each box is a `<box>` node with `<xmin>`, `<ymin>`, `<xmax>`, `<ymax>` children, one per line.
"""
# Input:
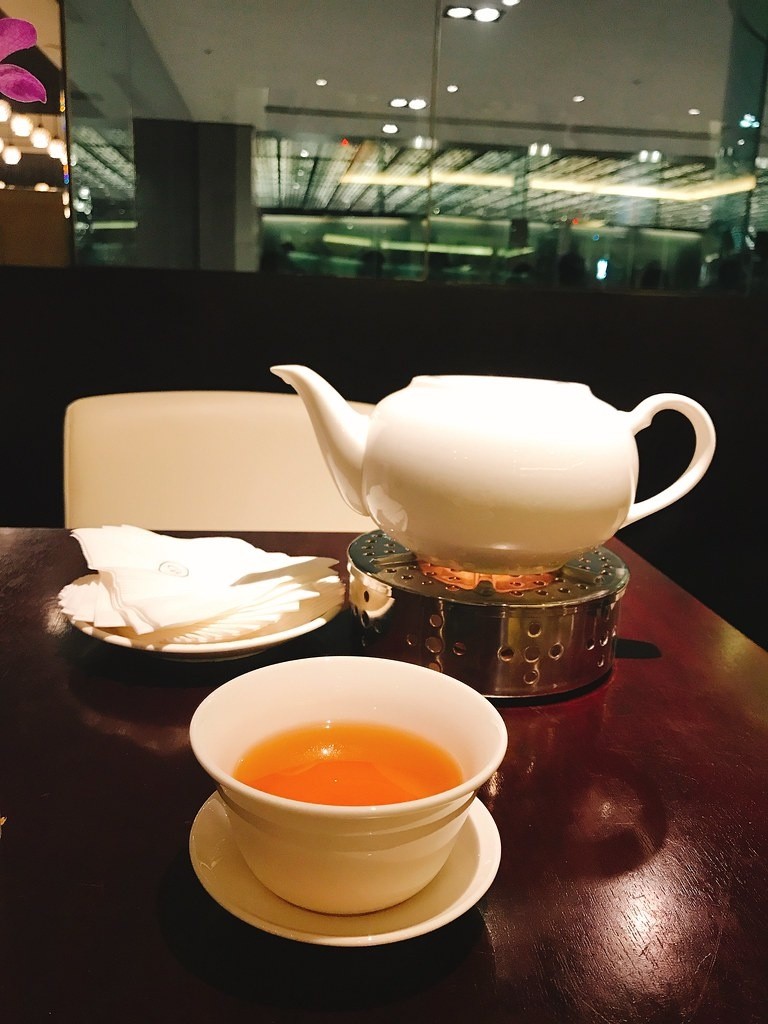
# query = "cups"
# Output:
<box><xmin>188</xmin><ymin>656</ymin><xmax>509</xmax><ymax>916</ymax></box>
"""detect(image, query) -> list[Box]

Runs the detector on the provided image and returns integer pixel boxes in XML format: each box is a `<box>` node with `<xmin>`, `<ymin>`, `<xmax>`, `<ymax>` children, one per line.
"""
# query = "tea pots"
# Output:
<box><xmin>269</xmin><ymin>363</ymin><xmax>716</xmax><ymax>574</ymax></box>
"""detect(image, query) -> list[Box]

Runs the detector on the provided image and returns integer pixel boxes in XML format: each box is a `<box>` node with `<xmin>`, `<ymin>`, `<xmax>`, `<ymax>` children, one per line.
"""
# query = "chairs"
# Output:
<box><xmin>63</xmin><ymin>391</ymin><xmax>384</xmax><ymax>533</ymax></box>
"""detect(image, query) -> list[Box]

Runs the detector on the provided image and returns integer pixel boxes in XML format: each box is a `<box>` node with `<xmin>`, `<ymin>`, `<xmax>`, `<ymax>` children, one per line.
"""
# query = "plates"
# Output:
<box><xmin>187</xmin><ymin>790</ymin><xmax>502</xmax><ymax>948</ymax></box>
<box><xmin>60</xmin><ymin>573</ymin><xmax>345</xmax><ymax>663</ymax></box>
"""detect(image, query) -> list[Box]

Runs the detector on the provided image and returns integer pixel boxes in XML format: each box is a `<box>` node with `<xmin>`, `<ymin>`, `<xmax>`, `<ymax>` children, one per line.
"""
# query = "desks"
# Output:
<box><xmin>0</xmin><ymin>526</ymin><xmax>768</xmax><ymax>1024</ymax></box>
